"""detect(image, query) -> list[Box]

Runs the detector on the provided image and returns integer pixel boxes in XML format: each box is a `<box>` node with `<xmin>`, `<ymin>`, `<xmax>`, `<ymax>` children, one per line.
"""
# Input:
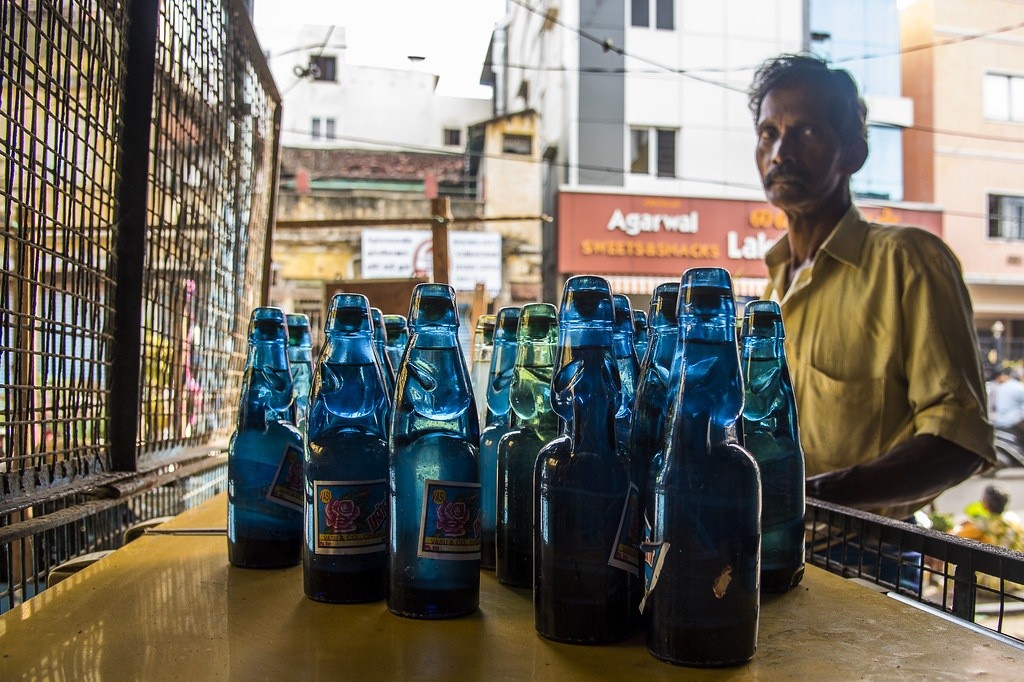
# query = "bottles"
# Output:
<box><xmin>304</xmin><ymin>292</ymin><xmax>390</xmax><ymax>605</ymax></box>
<box><xmin>612</xmin><ymin>293</ymin><xmax>642</xmax><ymax>456</ymax></box>
<box><xmin>532</xmin><ymin>275</ymin><xmax>646</xmax><ymax>647</ymax></box>
<box><xmin>369</xmin><ymin>306</ymin><xmax>396</xmax><ymax>402</ymax></box>
<box><xmin>632</xmin><ymin>309</ymin><xmax>648</xmax><ymax>364</ymax></box>
<box><xmin>731</xmin><ymin>300</ymin><xmax>806</xmax><ymax>600</ymax></box>
<box><xmin>383</xmin><ymin>314</ymin><xmax>410</xmax><ymax>379</ymax></box>
<box><xmin>479</xmin><ymin>306</ymin><xmax>520</xmax><ymax>572</ymax></box>
<box><xmin>496</xmin><ymin>302</ymin><xmax>560</xmax><ymax>588</ymax></box>
<box><xmin>389</xmin><ymin>282</ymin><xmax>482</xmax><ymax>621</ymax></box>
<box><xmin>227</xmin><ymin>306</ymin><xmax>305</xmax><ymax>571</ymax></box>
<box><xmin>631</xmin><ymin>282</ymin><xmax>680</xmax><ymax>463</ymax></box>
<box><xmin>469</xmin><ymin>313</ymin><xmax>497</xmax><ymax>436</ymax></box>
<box><xmin>645</xmin><ymin>266</ymin><xmax>762</xmax><ymax>667</ymax></box>
<box><xmin>284</xmin><ymin>312</ymin><xmax>314</xmax><ymax>432</ymax></box>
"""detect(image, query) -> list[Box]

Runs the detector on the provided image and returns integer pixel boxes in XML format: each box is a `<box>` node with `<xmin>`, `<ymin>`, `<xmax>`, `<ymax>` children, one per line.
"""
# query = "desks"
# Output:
<box><xmin>0</xmin><ymin>491</ymin><xmax>1024</xmax><ymax>682</ymax></box>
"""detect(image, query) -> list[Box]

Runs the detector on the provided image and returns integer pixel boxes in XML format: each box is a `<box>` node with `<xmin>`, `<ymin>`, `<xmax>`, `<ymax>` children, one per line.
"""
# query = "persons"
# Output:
<box><xmin>747</xmin><ymin>50</ymin><xmax>1024</xmax><ymax>596</ymax></box>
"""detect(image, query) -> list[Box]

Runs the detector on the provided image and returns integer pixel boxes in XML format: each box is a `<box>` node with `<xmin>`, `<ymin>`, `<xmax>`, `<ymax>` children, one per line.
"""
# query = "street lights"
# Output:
<box><xmin>989</xmin><ymin>320</ymin><xmax>1008</xmax><ymax>366</ymax></box>
